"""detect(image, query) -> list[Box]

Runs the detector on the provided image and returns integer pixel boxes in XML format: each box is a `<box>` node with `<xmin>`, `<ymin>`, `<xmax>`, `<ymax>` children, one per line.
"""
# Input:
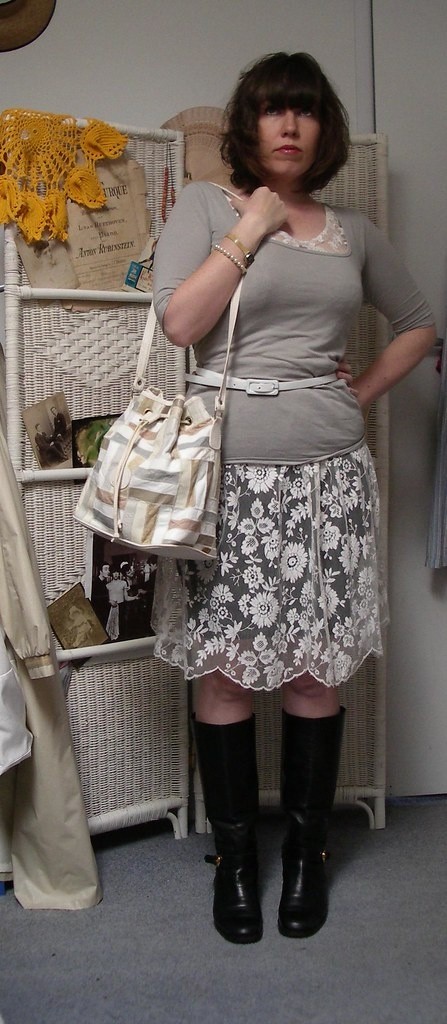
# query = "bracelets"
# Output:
<box><xmin>224</xmin><ymin>231</ymin><xmax>255</xmax><ymax>266</ymax></box>
<box><xmin>213</xmin><ymin>245</ymin><xmax>248</xmax><ymax>275</ymax></box>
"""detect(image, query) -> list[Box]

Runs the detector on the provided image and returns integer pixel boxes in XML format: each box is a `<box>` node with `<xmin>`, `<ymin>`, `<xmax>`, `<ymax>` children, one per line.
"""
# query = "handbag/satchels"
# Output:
<box><xmin>70</xmin><ymin>180</ymin><xmax>244</xmax><ymax>560</ymax></box>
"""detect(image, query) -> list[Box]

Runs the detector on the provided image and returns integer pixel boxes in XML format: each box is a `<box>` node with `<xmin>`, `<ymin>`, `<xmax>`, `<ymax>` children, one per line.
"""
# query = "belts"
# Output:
<box><xmin>184</xmin><ymin>367</ymin><xmax>339</xmax><ymax>395</ymax></box>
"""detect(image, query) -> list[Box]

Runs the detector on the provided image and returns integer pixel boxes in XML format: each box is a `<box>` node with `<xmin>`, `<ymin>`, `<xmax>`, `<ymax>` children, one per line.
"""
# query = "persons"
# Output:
<box><xmin>150</xmin><ymin>49</ymin><xmax>440</xmax><ymax>944</ymax></box>
<box><xmin>68</xmin><ymin>605</ymin><xmax>93</xmax><ymax>647</ymax></box>
<box><xmin>94</xmin><ymin>557</ymin><xmax>158</xmax><ymax>640</ymax></box>
<box><xmin>34</xmin><ymin>405</ymin><xmax>69</xmax><ymax>463</ymax></box>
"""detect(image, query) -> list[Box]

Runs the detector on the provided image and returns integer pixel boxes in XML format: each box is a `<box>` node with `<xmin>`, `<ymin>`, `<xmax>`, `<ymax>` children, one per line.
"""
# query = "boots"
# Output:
<box><xmin>277</xmin><ymin>705</ymin><xmax>345</xmax><ymax>938</ymax></box>
<box><xmin>191</xmin><ymin>711</ymin><xmax>264</xmax><ymax>944</ymax></box>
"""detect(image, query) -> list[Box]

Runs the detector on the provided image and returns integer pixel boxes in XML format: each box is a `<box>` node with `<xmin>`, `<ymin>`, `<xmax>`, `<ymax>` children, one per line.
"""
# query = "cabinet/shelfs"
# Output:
<box><xmin>194</xmin><ymin>132</ymin><xmax>387</xmax><ymax>836</ymax></box>
<box><xmin>0</xmin><ymin>110</ymin><xmax>188</xmax><ymax>883</ymax></box>
